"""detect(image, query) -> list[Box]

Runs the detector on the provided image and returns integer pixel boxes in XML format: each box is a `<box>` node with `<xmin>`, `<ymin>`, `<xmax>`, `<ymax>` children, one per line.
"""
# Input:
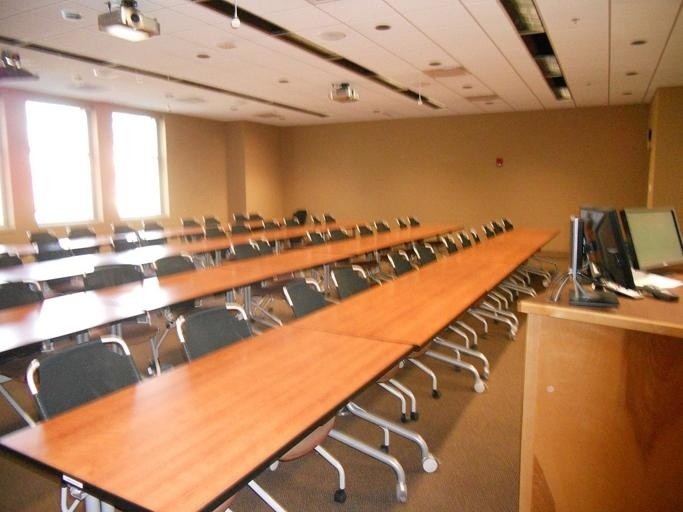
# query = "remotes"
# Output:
<box><xmin>646</xmin><ymin>283</ymin><xmax>679</xmax><ymax>301</ymax></box>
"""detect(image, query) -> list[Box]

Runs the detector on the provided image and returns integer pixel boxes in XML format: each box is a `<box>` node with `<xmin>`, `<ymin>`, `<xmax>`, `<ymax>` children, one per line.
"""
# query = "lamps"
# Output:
<box><xmin>232</xmin><ymin>0</ymin><xmax>240</xmax><ymax>28</ymax></box>
<box><xmin>417</xmin><ymin>91</ymin><xmax>423</xmax><ymax>106</ymax></box>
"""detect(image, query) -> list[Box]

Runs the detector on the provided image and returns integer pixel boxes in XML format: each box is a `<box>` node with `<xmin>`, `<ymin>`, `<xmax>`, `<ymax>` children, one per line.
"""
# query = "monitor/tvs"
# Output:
<box><xmin>569</xmin><ymin>207</ymin><xmax>635</xmax><ymax>306</ymax></box>
<box><xmin>620</xmin><ymin>204</ymin><xmax>683</xmax><ymax>270</ymax></box>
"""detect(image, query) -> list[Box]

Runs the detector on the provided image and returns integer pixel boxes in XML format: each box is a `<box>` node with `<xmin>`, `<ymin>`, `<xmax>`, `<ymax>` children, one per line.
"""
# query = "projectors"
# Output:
<box><xmin>97</xmin><ymin>8</ymin><xmax>160</xmax><ymax>43</ymax></box>
<box><xmin>330</xmin><ymin>87</ymin><xmax>358</xmax><ymax>101</ymax></box>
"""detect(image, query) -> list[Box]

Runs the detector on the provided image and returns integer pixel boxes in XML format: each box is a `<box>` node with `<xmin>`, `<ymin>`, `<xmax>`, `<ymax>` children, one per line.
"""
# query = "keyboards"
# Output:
<box><xmin>606</xmin><ymin>281</ymin><xmax>642</xmax><ymax>300</ymax></box>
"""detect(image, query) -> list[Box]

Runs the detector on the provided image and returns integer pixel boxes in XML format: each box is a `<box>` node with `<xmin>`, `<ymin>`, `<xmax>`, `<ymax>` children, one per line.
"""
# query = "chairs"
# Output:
<box><xmin>0</xmin><ymin>210</ymin><xmax>558</xmax><ymax>512</ymax></box>
